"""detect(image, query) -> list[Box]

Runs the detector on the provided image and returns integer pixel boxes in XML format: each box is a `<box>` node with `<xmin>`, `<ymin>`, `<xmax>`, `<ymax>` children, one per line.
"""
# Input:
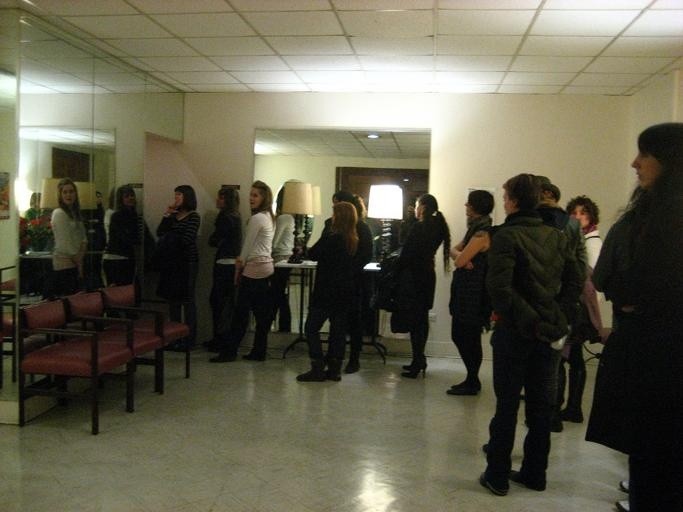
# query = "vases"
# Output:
<box><xmin>30</xmin><ymin>238</ymin><xmax>48</xmax><ymax>251</ymax></box>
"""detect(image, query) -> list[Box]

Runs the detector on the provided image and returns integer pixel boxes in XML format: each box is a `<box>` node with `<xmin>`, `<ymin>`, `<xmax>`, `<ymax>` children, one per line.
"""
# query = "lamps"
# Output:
<box><xmin>367</xmin><ymin>183</ymin><xmax>404</xmax><ymax>264</ymax></box>
<box><xmin>305</xmin><ymin>186</ymin><xmax>321</xmax><ymax>259</ymax></box>
<box><xmin>281</xmin><ymin>182</ymin><xmax>311</xmax><ymax>263</ymax></box>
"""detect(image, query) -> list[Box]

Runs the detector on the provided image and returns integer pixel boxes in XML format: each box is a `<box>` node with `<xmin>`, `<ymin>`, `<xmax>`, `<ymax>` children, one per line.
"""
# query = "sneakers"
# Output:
<box><xmin>242</xmin><ymin>351</ymin><xmax>266</xmax><ymax>362</ymax></box>
<box><xmin>208</xmin><ymin>353</ymin><xmax>237</xmax><ymax>362</ymax></box>
<box><xmin>524</xmin><ymin>418</ymin><xmax>563</xmax><ymax>433</ymax></box>
<box><xmin>482</xmin><ymin>442</ymin><xmax>489</xmax><ymax>451</ymax></box>
<box><xmin>446</xmin><ymin>385</ymin><xmax>477</xmax><ymax>396</ymax></box>
<box><xmin>560</xmin><ymin>408</ymin><xmax>583</xmax><ymax>423</ymax></box>
<box><xmin>619</xmin><ymin>480</ymin><xmax>629</xmax><ymax>492</ymax></box>
<box><xmin>479</xmin><ymin>471</ymin><xmax>509</xmax><ymax>496</ymax></box>
<box><xmin>510</xmin><ymin>470</ymin><xmax>547</xmax><ymax>491</ymax></box>
<box><xmin>344</xmin><ymin>359</ymin><xmax>360</xmax><ymax>373</ymax></box>
<box><xmin>296</xmin><ymin>369</ymin><xmax>327</xmax><ymax>382</ymax></box>
<box><xmin>325</xmin><ymin>366</ymin><xmax>342</xmax><ymax>382</ymax></box>
<box><xmin>615</xmin><ymin>499</ymin><xmax>630</xmax><ymax>510</ymax></box>
<box><xmin>451</xmin><ymin>381</ymin><xmax>481</xmax><ymax>391</ymax></box>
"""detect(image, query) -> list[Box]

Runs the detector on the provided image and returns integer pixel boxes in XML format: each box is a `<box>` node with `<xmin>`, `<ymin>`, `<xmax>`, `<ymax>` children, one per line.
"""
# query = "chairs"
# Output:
<box><xmin>94</xmin><ymin>284</ymin><xmax>193</xmax><ymax>392</ymax></box>
<box><xmin>19</xmin><ymin>297</ymin><xmax>132</xmax><ymax>435</ymax></box>
<box><xmin>0</xmin><ymin>264</ymin><xmax>18</xmax><ymax>390</ymax></box>
<box><xmin>55</xmin><ymin>291</ymin><xmax>164</xmax><ymax>394</ymax></box>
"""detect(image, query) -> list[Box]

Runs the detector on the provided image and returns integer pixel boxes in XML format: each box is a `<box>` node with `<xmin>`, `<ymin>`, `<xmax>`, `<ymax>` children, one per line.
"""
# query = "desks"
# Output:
<box><xmin>217</xmin><ymin>257</ymin><xmax>387</xmax><ymax>366</ymax></box>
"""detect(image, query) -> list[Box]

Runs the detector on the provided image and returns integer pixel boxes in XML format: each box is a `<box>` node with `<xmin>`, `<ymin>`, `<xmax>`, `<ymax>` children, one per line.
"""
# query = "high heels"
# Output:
<box><xmin>403</xmin><ymin>357</ymin><xmax>426</xmax><ymax>369</ymax></box>
<box><xmin>401</xmin><ymin>362</ymin><xmax>427</xmax><ymax>378</ymax></box>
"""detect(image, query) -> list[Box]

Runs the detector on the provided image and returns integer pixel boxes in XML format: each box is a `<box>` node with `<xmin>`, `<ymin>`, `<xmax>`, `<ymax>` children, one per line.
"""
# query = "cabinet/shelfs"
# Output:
<box><xmin>19</xmin><ymin>257</ymin><xmax>53</xmax><ymax>295</ymax></box>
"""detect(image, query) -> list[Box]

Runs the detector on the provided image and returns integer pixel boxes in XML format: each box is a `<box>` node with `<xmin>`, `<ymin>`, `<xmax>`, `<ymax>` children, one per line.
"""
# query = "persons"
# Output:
<box><xmin>208</xmin><ymin>180</ymin><xmax>277</xmax><ymax>364</ymax></box>
<box><xmin>295</xmin><ymin>201</ymin><xmax>358</xmax><ymax>383</ymax></box>
<box><xmin>589</xmin><ymin>121</ymin><xmax>683</xmax><ymax>512</ymax></box>
<box><xmin>389</xmin><ymin>195</ymin><xmax>451</xmax><ymax>379</ymax></box>
<box><xmin>94</xmin><ymin>192</ymin><xmax>105</xmax><ymax>288</ymax></box>
<box><xmin>445</xmin><ymin>189</ymin><xmax>495</xmax><ymax>396</ymax></box>
<box><xmin>156</xmin><ymin>185</ymin><xmax>201</xmax><ymax>352</ymax></box>
<box><xmin>103</xmin><ymin>186</ymin><xmax>114</xmax><ymax>285</ymax></box>
<box><xmin>558</xmin><ymin>195</ymin><xmax>603</xmax><ymax>423</ymax></box>
<box><xmin>267</xmin><ymin>185</ymin><xmax>295</xmax><ymax>334</ymax></box>
<box><xmin>108</xmin><ymin>185</ymin><xmax>141</xmax><ymax>319</ymax></box>
<box><xmin>25</xmin><ymin>192</ymin><xmax>48</xmax><ymax>296</ymax></box>
<box><xmin>482</xmin><ymin>175</ymin><xmax>588</xmax><ymax>455</ymax></box>
<box><xmin>52</xmin><ymin>178</ymin><xmax>87</xmax><ymax>295</ymax></box>
<box><xmin>323</xmin><ymin>192</ymin><xmax>373</xmax><ymax>375</ymax></box>
<box><xmin>524</xmin><ymin>184</ymin><xmax>570</xmax><ymax>433</ymax></box>
<box><xmin>202</xmin><ymin>187</ymin><xmax>241</xmax><ymax>351</ymax></box>
<box><xmin>478</xmin><ymin>173</ymin><xmax>584</xmax><ymax>497</ymax></box>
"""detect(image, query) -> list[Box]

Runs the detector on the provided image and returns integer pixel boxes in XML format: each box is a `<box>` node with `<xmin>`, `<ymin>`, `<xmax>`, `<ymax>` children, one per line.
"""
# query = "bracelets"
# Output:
<box><xmin>234</xmin><ymin>269</ymin><xmax>241</xmax><ymax>272</ymax></box>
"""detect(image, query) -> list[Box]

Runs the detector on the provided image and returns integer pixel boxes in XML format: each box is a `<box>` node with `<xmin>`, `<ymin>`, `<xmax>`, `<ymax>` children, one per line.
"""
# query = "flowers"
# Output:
<box><xmin>20</xmin><ymin>207</ymin><xmax>55</xmax><ymax>240</ymax></box>
